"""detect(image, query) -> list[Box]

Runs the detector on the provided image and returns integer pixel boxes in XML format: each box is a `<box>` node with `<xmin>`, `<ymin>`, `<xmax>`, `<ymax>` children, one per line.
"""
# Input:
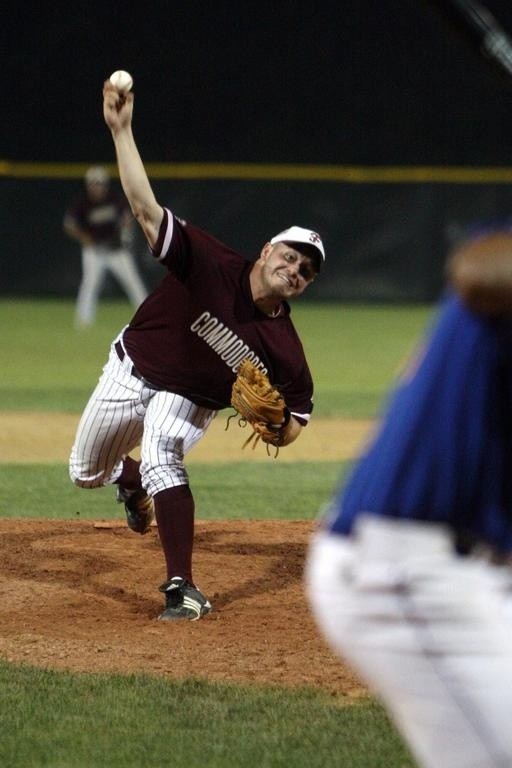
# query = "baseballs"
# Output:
<box><xmin>110</xmin><ymin>71</ymin><xmax>133</xmax><ymax>94</ymax></box>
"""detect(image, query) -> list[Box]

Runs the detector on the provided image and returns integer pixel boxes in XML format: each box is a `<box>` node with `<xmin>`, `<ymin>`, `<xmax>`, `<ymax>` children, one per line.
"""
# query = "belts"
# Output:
<box><xmin>114</xmin><ymin>341</ymin><xmax>163</xmax><ymax>392</ymax></box>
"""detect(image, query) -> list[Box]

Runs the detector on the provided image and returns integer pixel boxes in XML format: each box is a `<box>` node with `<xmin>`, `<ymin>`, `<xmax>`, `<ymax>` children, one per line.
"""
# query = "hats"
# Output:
<box><xmin>269</xmin><ymin>226</ymin><xmax>327</xmax><ymax>262</ymax></box>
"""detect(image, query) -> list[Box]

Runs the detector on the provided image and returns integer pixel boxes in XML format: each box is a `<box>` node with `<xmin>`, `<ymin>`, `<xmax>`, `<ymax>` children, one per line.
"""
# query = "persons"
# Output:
<box><xmin>302</xmin><ymin>219</ymin><xmax>509</xmax><ymax>766</ymax></box>
<box><xmin>65</xmin><ymin>80</ymin><xmax>328</xmax><ymax>625</ymax></box>
<box><xmin>62</xmin><ymin>166</ymin><xmax>147</xmax><ymax>332</ymax></box>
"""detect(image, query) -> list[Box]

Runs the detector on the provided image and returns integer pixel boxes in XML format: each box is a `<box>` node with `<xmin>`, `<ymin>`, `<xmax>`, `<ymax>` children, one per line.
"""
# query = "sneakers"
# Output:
<box><xmin>158</xmin><ymin>575</ymin><xmax>213</xmax><ymax>623</ymax></box>
<box><xmin>116</xmin><ymin>483</ymin><xmax>155</xmax><ymax>533</ymax></box>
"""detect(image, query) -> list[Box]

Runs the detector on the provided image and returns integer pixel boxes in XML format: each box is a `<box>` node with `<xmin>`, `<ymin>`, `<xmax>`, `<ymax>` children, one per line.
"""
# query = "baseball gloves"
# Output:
<box><xmin>231</xmin><ymin>357</ymin><xmax>292</xmax><ymax>446</ymax></box>
